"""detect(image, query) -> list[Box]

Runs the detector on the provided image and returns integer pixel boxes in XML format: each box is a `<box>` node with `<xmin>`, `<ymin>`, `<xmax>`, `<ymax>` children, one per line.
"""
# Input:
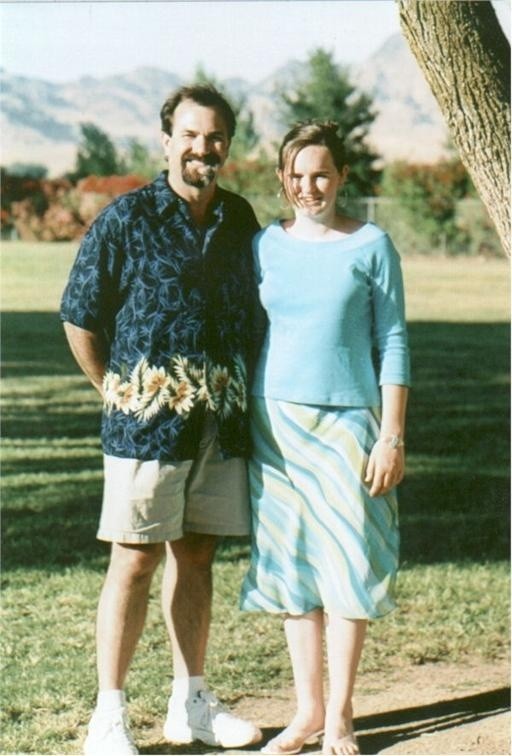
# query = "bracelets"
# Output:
<box><xmin>378</xmin><ymin>427</ymin><xmax>405</xmax><ymax>449</ymax></box>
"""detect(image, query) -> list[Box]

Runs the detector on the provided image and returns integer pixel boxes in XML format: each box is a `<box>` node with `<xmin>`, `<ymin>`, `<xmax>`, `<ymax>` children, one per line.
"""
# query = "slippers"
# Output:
<box><xmin>260</xmin><ymin>728</ymin><xmax>361</xmax><ymax>755</ymax></box>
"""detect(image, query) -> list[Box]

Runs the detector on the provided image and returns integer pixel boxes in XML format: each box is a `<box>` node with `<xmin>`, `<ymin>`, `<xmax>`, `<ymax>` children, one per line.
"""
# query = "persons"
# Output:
<box><xmin>235</xmin><ymin>120</ymin><xmax>416</xmax><ymax>753</ymax></box>
<box><xmin>57</xmin><ymin>82</ymin><xmax>266</xmax><ymax>756</ymax></box>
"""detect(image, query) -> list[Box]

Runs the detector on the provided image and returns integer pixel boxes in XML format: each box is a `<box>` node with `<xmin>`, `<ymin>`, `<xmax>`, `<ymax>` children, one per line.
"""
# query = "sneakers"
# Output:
<box><xmin>163</xmin><ymin>691</ymin><xmax>262</xmax><ymax>748</ymax></box>
<box><xmin>84</xmin><ymin>706</ymin><xmax>139</xmax><ymax>755</ymax></box>
